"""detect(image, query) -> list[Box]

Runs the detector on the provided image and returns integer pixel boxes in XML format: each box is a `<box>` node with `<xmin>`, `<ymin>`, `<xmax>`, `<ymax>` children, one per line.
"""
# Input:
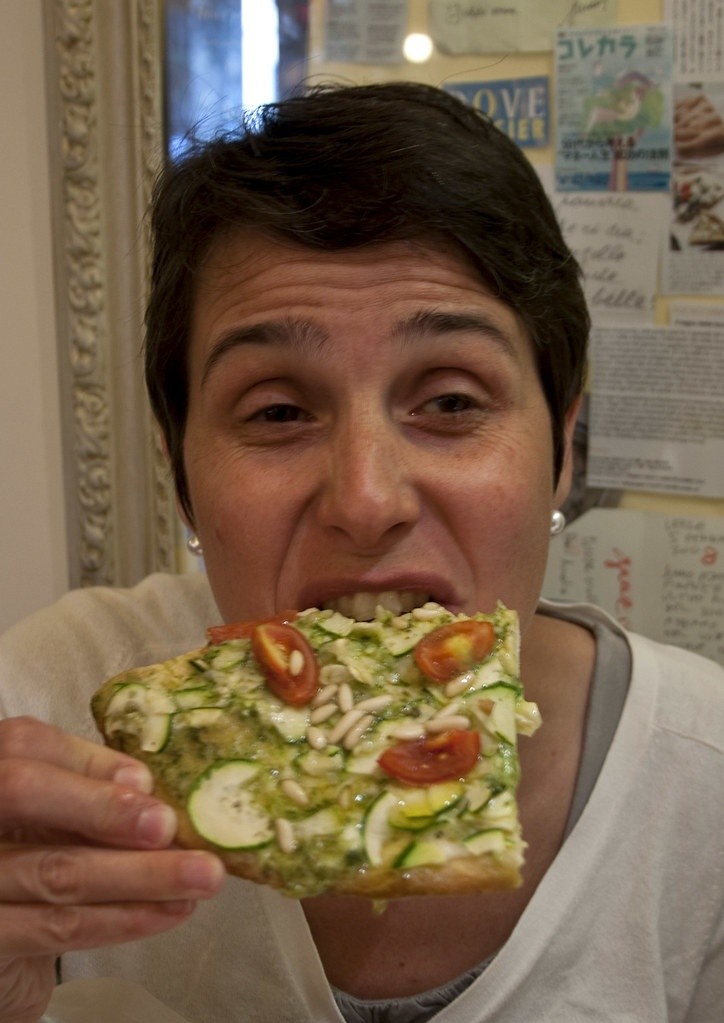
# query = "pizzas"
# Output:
<box><xmin>87</xmin><ymin>598</ymin><xmax>547</xmax><ymax>904</ymax></box>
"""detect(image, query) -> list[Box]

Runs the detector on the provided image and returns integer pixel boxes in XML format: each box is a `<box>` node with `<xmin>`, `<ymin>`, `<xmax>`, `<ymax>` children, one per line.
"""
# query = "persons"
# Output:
<box><xmin>1</xmin><ymin>76</ymin><xmax>724</xmax><ymax>1023</ymax></box>
